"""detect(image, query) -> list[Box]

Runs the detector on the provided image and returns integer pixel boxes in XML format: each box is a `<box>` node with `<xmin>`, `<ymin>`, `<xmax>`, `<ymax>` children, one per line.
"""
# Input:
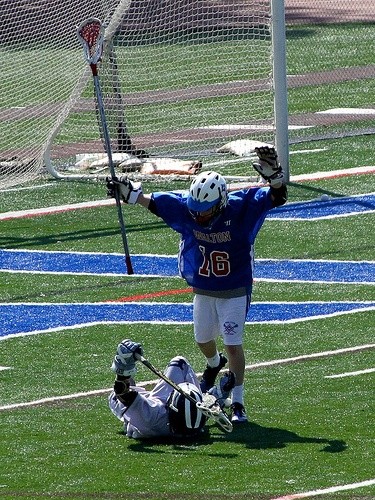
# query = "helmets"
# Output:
<box><xmin>186</xmin><ymin>171</ymin><xmax>228</xmax><ymax>224</ymax></box>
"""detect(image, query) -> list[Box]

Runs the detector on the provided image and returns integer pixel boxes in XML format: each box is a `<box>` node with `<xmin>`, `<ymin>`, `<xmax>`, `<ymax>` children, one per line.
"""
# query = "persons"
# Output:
<box><xmin>106</xmin><ymin>146</ymin><xmax>287</xmax><ymax>425</ymax></box>
<box><xmin>108</xmin><ymin>339</ymin><xmax>236</xmax><ymax>441</ymax></box>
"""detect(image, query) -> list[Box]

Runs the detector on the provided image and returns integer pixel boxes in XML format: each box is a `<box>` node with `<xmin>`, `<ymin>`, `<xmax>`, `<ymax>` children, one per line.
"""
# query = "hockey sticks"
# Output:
<box><xmin>134</xmin><ymin>352</ymin><xmax>233</xmax><ymax>433</ymax></box>
<box><xmin>75</xmin><ymin>17</ymin><xmax>134</xmax><ymax>277</ymax></box>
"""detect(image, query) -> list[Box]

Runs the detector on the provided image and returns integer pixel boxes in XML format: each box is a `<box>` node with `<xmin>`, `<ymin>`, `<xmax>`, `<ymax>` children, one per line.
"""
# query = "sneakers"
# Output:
<box><xmin>229</xmin><ymin>402</ymin><xmax>250</xmax><ymax>424</ymax></box>
<box><xmin>197</xmin><ymin>353</ymin><xmax>228</xmax><ymax>383</ymax></box>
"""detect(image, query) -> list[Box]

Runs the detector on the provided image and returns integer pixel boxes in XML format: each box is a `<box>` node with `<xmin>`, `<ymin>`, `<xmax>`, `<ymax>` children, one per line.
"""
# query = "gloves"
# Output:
<box><xmin>104</xmin><ymin>176</ymin><xmax>143</xmax><ymax>205</ymax></box>
<box><xmin>111</xmin><ymin>338</ymin><xmax>144</xmax><ymax>375</ymax></box>
<box><xmin>221</xmin><ymin>371</ymin><xmax>236</xmax><ymax>392</ymax></box>
<box><xmin>253</xmin><ymin>145</ymin><xmax>285</xmax><ymax>185</ymax></box>
